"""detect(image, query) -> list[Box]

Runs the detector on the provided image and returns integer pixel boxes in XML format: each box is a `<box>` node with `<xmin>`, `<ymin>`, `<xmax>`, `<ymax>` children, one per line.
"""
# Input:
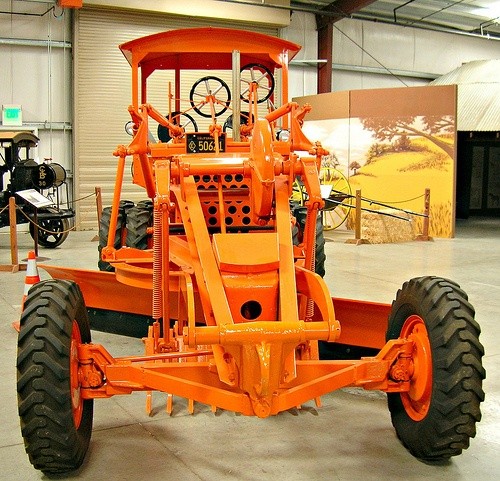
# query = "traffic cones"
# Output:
<box><xmin>12</xmin><ymin>251</ymin><xmax>40</xmax><ymax>333</ymax></box>
